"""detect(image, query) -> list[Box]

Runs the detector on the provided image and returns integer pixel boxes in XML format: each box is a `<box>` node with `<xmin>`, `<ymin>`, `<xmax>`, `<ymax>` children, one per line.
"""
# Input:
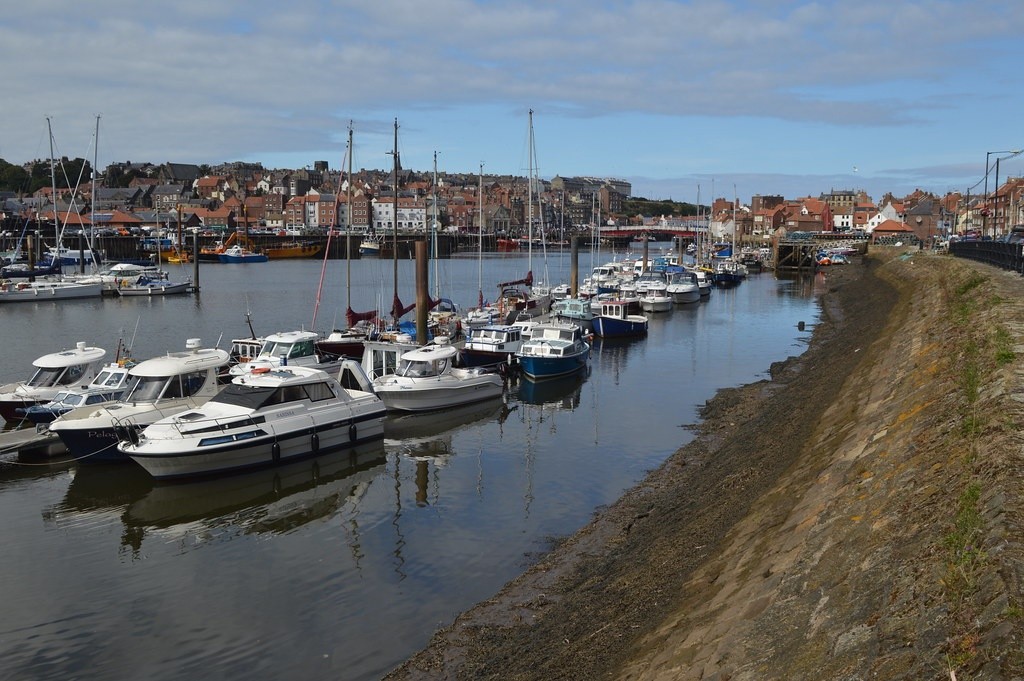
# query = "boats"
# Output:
<box><xmin>0</xmin><ymin>105</ymin><xmax>870</xmax><ymax>484</ymax></box>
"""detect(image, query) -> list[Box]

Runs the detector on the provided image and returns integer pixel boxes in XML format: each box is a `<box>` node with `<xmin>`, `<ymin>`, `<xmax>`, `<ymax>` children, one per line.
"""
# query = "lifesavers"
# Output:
<box><xmin>252</xmin><ymin>368</ymin><xmax>270</xmax><ymax>373</ymax></box>
<box><xmin>122</xmin><ymin>279</ymin><xmax>128</xmax><ymax>286</ymax></box>
